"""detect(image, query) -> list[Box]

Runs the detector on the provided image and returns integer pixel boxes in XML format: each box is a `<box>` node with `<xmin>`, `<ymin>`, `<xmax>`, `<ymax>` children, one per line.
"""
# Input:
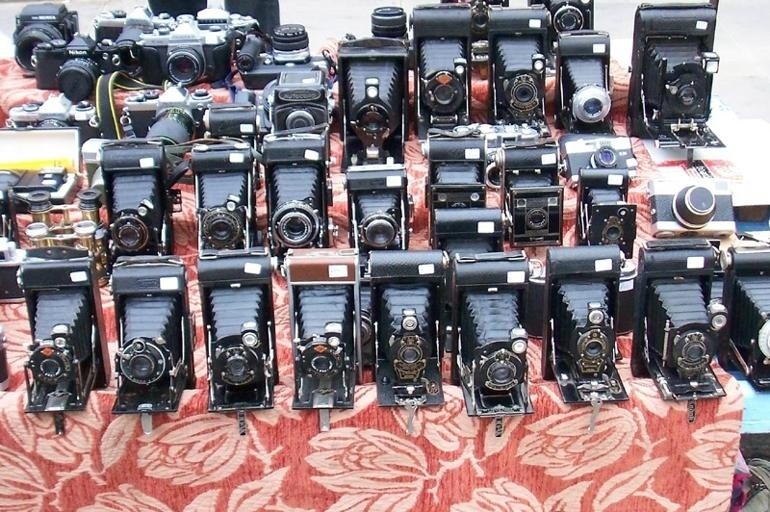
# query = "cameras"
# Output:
<box><xmin>136</xmin><ymin>8</ymin><xmax>235</xmax><ymax>88</ymax></box>
<box><xmin>0</xmin><ymin>330</ymin><xmax>11</xmax><ymax>393</ymax></box>
<box><xmin>33</xmin><ymin>33</ymin><xmax>115</xmax><ymax>104</ymax></box>
<box><xmin>93</xmin><ymin>7</ymin><xmax>176</xmax><ymax>42</ymax></box>
<box><xmin>368</xmin><ymin>251</ymin><xmax>450</xmax><ymax>408</ymax></box>
<box><xmin>340</xmin><ymin>37</ymin><xmax>409</xmax><ymax>173</ymax></box>
<box><xmin>0</xmin><ymin>163</ymin><xmax>78</xmax><ymax>212</ymax></box>
<box><xmin>449</xmin><ymin>249</ymin><xmax>536</xmax><ymax>416</ymax></box>
<box><xmin>281</xmin><ymin>246</ymin><xmax>358</xmax><ymax>410</ymax></box>
<box><xmin>196</xmin><ymin>249</ymin><xmax>280</xmax><ymax>412</ymax></box>
<box><xmin>238</xmin><ymin>22</ymin><xmax>334</xmax><ymax>96</ymax></box>
<box><xmin>625</xmin><ymin>0</ymin><xmax>726</xmax><ymax>152</ymax></box>
<box><xmin>100</xmin><ymin>145</ymin><xmax>174</xmax><ymax>283</ymax></box>
<box><xmin>629</xmin><ymin>240</ymin><xmax>728</xmax><ymax>402</ymax></box>
<box><xmin>24</xmin><ymin>220</ymin><xmax>96</xmax><ymax>251</ymax></box>
<box><xmin>24</xmin><ymin>187</ymin><xmax>99</xmax><ymax>226</ymax></box>
<box><xmin>82</xmin><ymin>137</ymin><xmax>106</xmax><ymax>208</ymax></box>
<box><xmin>369</xmin><ymin>6</ymin><xmax>412</xmax><ymax>65</ymax></box>
<box><xmin>6</xmin><ymin>93</ymin><xmax>101</xmax><ymax>144</ymax></box>
<box><xmin>92</xmin><ymin>18</ymin><xmax>175</xmax><ymax>79</ymax></box>
<box><xmin>261</xmin><ymin>68</ymin><xmax>330</xmax><ymax>138</ymax></box>
<box><xmin>562</xmin><ymin>133</ymin><xmax>638</xmax><ymax>188</ymax></box>
<box><xmin>191</xmin><ymin>141</ymin><xmax>263</xmax><ymax>260</ymax></box>
<box><xmin>260</xmin><ymin>131</ymin><xmax>332</xmax><ymax>271</ymax></box>
<box><xmin>201</xmin><ymin>103</ymin><xmax>262</xmax><ymax>141</ymax></box>
<box><xmin>411</xmin><ymin>5</ymin><xmax>475</xmax><ymax>138</ymax></box>
<box><xmin>0</xmin><ymin>236</ymin><xmax>26</xmax><ymax>303</ymax></box>
<box><xmin>451</xmin><ymin>124</ymin><xmax>551</xmax><ymax>189</ymax></box>
<box><xmin>651</xmin><ymin>185</ymin><xmax>737</xmax><ymax>240</ymax></box>
<box><xmin>721</xmin><ymin>244</ymin><xmax>770</xmax><ymax>394</ymax></box>
<box><xmin>527</xmin><ymin>1</ymin><xmax>595</xmax><ymax>38</ymax></box>
<box><xmin>422</xmin><ymin>136</ymin><xmax>489</xmax><ymax>208</ymax></box>
<box><xmin>12</xmin><ymin>1</ymin><xmax>80</xmax><ymax>78</ymax></box>
<box><xmin>487</xmin><ymin>10</ymin><xmax>548</xmax><ymax>127</ymax></box>
<box><xmin>119</xmin><ymin>88</ymin><xmax>213</xmax><ymax>146</ymax></box>
<box><xmin>542</xmin><ymin>245</ymin><xmax>629</xmax><ymax>405</ymax></box>
<box><xmin>110</xmin><ymin>254</ymin><xmax>194</xmax><ymax>417</ymax></box>
<box><xmin>439</xmin><ymin>0</ymin><xmax>512</xmax><ymax>63</ymax></box>
<box><xmin>16</xmin><ymin>249</ymin><xmax>112</xmax><ymax>413</ymax></box>
<box><xmin>576</xmin><ymin>169</ymin><xmax>638</xmax><ymax>259</ymax></box>
<box><xmin>270</xmin><ymin>85</ymin><xmax>329</xmax><ymax>136</ymax></box>
<box><xmin>556</xmin><ymin>32</ymin><xmax>616</xmax><ymax>137</ymax></box>
<box><xmin>345</xmin><ymin>167</ymin><xmax>414</xmax><ymax>278</ymax></box>
